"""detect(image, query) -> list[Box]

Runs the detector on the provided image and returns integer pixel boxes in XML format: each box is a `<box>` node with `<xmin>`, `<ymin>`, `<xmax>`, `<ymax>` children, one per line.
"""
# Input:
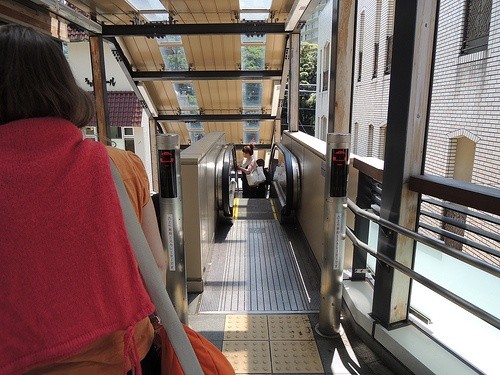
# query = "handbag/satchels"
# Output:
<box><xmin>154</xmin><ymin>322</ymin><xmax>236</xmax><ymax>375</ymax></box>
<box><xmin>246</xmin><ymin>159</ymin><xmax>267</xmax><ymax>186</ymax></box>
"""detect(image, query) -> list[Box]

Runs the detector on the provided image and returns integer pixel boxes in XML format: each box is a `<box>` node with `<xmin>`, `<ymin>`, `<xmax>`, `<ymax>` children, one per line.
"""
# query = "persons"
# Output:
<box><xmin>0</xmin><ymin>22</ymin><xmax>167</xmax><ymax>374</ymax></box>
<box><xmin>256</xmin><ymin>159</ymin><xmax>270</xmax><ymax>198</ymax></box>
<box><xmin>237</xmin><ymin>144</ymin><xmax>257</xmax><ymax>198</ymax></box>
